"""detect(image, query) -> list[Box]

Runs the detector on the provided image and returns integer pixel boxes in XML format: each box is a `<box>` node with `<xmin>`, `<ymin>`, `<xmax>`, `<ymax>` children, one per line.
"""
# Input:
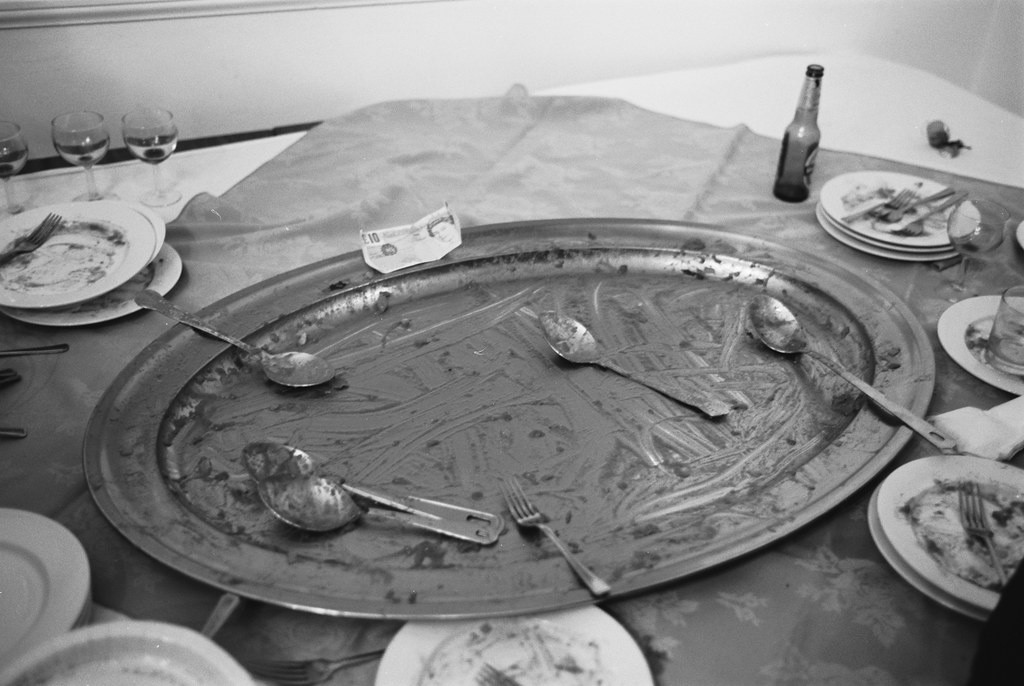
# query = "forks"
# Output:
<box><xmin>255</xmin><ymin>646</ymin><xmax>387</xmax><ymax>686</ymax></box>
<box><xmin>0</xmin><ymin>211</ymin><xmax>62</xmax><ymax>266</ymax></box>
<box><xmin>502</xmin><ymin>472</ymin><xmax>614</xmax><ymax>597</ymax></box>
<box><xmin>953</xmin><ymin>479</ymin><xmax>1010</xmax><ymax>598</ymax></box>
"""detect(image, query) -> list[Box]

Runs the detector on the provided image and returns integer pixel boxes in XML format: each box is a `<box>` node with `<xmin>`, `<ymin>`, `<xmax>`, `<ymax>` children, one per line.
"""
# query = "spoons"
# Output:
<box><xmin>242</xmin><ymin>439</ymin><xmax>506</xmax><ymax>548</ymax></box>
<box><xmin>535</xmin><ymin>305</ymin><xmax>729</xmax><ymax>418</ymax></box>
<box><xmin>745</xmin><ymin>292</ymin><xmax>958</xmax><ymax>452</ymax></box>
<box><xmin>132</xmin><ymin>286</ymin><xmax>337</xmax><ymax>390</ymax></box>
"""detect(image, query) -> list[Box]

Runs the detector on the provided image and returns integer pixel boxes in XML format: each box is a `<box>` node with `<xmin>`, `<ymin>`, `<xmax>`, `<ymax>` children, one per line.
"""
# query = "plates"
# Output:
<box><xmin>81</xmin><ymin>213</ymin><xmax>940</xmax><ymax>626</ymax></box>
<box><xmin>813</xmin><ymin>163</ymin><xmax>982</xmax><ymax>261</ymax></box>
<box><xmin>374</xmin><ymin>601</ymin><xmax>657</xmax><ymax>685</ymax></box>
<box><xmin>867</xmin><ymin>454</ymin><xmax>1024</xmax><ymax>624</ymax></box>
<box><xmin>936</xmin><ymin>290</ymin><xmax>1024</xmax><ymax>396</ymax></box>
<box><xmin>1</xmin><ymin>500</ymin><xmax>260</xmax><ymax>686</ymax></box>
<box><xmin>1</xmin><ymin>198</ymin><xmax>186</xmax><ymax>330</ymax></box>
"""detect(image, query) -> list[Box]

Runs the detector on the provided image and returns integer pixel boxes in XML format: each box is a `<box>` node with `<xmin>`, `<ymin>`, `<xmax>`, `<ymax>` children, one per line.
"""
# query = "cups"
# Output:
<box><xmin>984</xmin><ymin>284</ymin><xmax>1023</xmax><ymax>377</ymax></box>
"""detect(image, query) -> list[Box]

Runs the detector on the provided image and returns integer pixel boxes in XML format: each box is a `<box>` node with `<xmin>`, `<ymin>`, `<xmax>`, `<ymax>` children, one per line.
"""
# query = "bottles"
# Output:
<box><xmin>773</xmin><ymin>62</ymin><xmax>824</xmax><ymax>202</ymax></box>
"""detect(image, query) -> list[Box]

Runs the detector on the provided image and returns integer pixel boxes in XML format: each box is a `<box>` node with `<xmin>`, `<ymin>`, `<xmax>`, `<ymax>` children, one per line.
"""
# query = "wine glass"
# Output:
<box><xmin>124</xmin><ymin>106</ymin><xmax>184</xmax><ymax>207</ymax></box>
<box><xmin>936</xmin><ymin>197</ymin><xmax>1013</xmax><ymax>307</ymax></box>
<box><xmin>1</xmin><ymin>120</ymin><xmax>32</xmax><ymax>216</ymax></box>
<box><xmin>51</xmin><ymin>109</ymin><xmax>118</xmax><ymax>207</ymax></box>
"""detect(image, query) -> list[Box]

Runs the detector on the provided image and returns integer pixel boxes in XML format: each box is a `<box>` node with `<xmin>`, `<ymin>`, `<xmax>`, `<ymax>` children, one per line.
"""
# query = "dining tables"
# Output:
<box><xmin>0</xmin><ymin>51</ymin><xmax>1024</xmax><ymax>685</ymax></box>
